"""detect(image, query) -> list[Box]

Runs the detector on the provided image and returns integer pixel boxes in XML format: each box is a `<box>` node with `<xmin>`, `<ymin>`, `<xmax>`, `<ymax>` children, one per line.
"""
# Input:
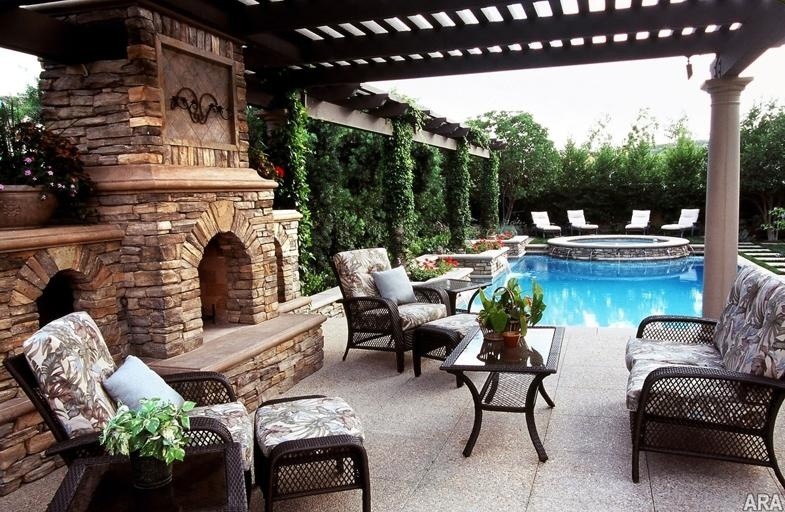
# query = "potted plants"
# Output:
<box><xmin>477</xmin><ymin>278</ymin><xmax>547</xmax><ymax>331</ymax></box>
<box><xmin>98</xmin><ymin>397</ymin><xmax>198</xmax><ymax>491</ymax></box>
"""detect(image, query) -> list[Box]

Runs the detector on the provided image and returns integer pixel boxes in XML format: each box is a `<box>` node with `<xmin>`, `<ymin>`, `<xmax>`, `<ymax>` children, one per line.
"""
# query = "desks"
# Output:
<box><xmin>46</xmin><ymin>442</ymin><xmax>248</xmax><ymax>512</ymax></box>
<box><xmin>422</xmin><ymin>277</ymin><xmax>492</xmax><ymax>315</ymax></box>
<box><xmin>439</xmin><ymin>321</ymin><xmax>565</xmax><ymax>462</ymax></box>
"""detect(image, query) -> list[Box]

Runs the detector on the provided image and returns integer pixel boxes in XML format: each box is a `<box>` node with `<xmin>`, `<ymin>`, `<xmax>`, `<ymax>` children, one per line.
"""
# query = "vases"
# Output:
<box><xmin>0</xmin><ymin>183</ymin><xmax>55</xmax><ymax>227</ymax></box>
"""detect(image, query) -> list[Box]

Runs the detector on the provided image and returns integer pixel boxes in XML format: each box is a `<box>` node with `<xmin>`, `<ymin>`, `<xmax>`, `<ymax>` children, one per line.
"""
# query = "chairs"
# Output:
<box><xmin>566</xmin><ymin>209</ymin><xmax>599</xmax><ymax>236</ymax></box>
<box><xmin>530</xmin><ymin>211</ymin><xmax>561</xmax><ymax>240</ymax></box>
<box><xmin>624</xmin><ymin>209</ymin><xmax>651</xmax><ymax>234</ymax></box>
<box><xmin>660</xmin><ymin>208</ymin><xmax>702</xmax><ymax>238</ymax></box>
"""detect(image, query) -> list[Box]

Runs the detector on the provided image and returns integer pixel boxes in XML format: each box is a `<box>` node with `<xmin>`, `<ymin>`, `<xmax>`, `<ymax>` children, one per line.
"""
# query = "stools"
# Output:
<box><xmin>253</xmin><ymin>394</ymin><xmax>371</xmax><ymax>512</ymax></box>
<box><xmin>412</xmin><ymin>312</ymin><xmax>480</xmax><ymax>388</ymax></box>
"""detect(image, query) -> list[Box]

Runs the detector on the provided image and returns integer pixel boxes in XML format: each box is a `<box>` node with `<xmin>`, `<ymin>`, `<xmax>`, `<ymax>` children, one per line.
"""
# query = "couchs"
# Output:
<box><xmin>625</xmin><ymin>264</ymin><xmax>785</xmax><ymax>494</ymax></box>
<box><xmin>327</xmin><ymin>247</ymin><xmax>451</xmax><ymax>374</ymax></box>
<box><xmin>2</xmin><ymin>309</ymin><xmax>254</xmax><ymax>512</ymax></box>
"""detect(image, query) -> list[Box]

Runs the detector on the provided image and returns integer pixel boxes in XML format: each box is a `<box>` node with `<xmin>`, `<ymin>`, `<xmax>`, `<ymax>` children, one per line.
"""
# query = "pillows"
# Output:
<box><xmin>372</xmin><ymin>264</ymin><xmax>418</xmax><ymax>307</ymax></box>
<box><xmin>102</xmin><ymin>354</ymin><xmax>188</xmax><ymax>418</ymax></box>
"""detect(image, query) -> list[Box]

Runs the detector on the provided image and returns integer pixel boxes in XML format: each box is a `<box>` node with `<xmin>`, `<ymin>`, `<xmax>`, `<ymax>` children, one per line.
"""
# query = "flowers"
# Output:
<box><xmin>0</xmin><ymin>102</ymin><xmax>99</xmax><ymax>210</ymax></box>
